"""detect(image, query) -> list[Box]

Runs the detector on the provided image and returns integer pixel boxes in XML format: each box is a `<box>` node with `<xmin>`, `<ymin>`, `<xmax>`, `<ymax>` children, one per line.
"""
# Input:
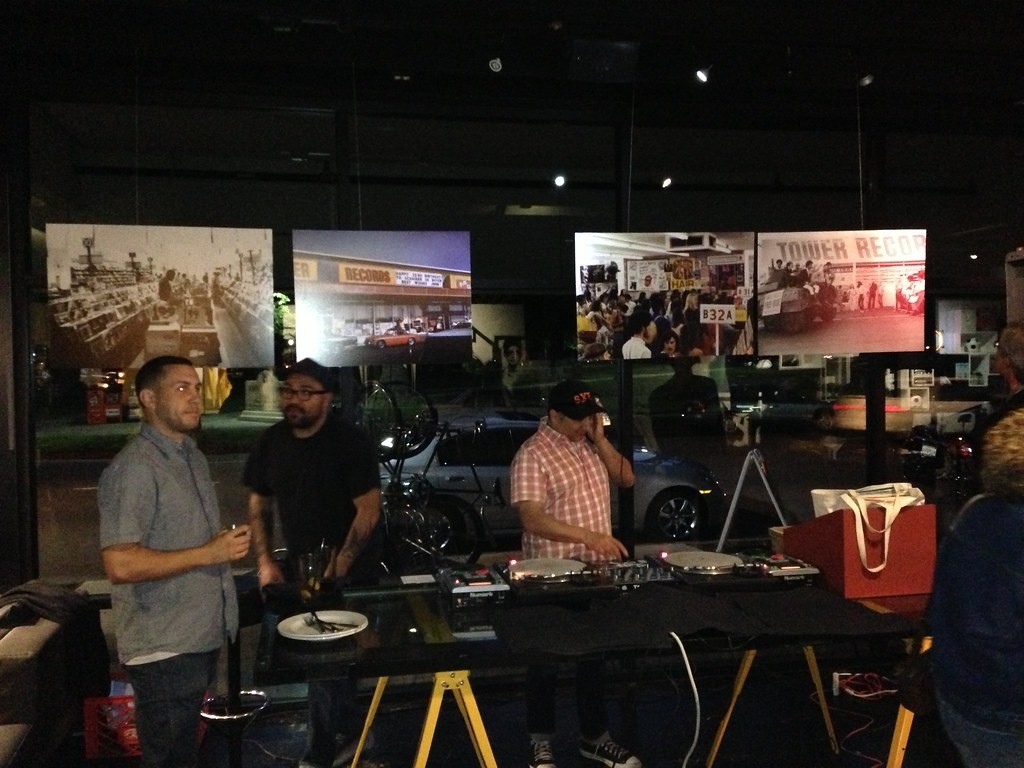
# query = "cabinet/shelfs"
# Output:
<box><xmin>898</xmin><ymin>369</ymin><xmax>935</xmax><ymax>410</ymax></box>
<box><xmin>936</xmin><ymin>300</ymin><xmax>1002</xmax><ymax>387</ymax></box>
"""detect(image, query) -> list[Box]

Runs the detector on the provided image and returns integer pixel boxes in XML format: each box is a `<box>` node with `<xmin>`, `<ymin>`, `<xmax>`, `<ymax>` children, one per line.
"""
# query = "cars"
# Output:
<box><xmin>376</xmin><ymin>411</ymin><xmax>728</xmax><ymax>548</ymax></box>
<box><xmin>365</xmin><ymin>327</ymin><xmax>422</xmax><ymax>351</ymax></box>
<box><xmin>718</xmin><ymin>384</ymin><xmax>837</xmax><ymax>439</ymax></box>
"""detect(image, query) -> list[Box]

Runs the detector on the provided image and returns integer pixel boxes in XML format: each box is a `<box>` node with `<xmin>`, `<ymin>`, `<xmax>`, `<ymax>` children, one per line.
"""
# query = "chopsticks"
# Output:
<box><xmin>311</xmin><ymin>609</ymin><xmax>357</xmax><ymax>633</ymax></box>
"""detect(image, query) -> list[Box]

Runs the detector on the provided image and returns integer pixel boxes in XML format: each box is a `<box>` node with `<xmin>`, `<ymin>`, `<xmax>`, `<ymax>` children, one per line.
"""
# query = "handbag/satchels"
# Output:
<box><xmin>811</xmin><ymin>480</ymin><xmax>927</xmax><ymax>573</ymax></box>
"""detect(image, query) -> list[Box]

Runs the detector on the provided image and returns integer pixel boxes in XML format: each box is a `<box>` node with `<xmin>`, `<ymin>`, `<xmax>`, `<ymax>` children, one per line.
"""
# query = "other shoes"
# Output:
<box><xmin>332</xmin><ymin>727</ymin><xmax>374</xmax><ymax>765</ymax></box>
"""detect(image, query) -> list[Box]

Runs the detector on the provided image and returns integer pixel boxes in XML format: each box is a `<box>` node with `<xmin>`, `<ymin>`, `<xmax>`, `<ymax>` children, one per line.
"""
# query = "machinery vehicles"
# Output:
<box><xmin>757</xmin><ymin>269</ymin><xmax>837</xmax><ymax>336</ymax></box>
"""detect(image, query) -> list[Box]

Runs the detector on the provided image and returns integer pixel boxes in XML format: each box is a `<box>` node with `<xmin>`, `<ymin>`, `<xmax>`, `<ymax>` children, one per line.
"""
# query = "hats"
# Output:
<box><xmin>278</xmin><ymin>358</ymin><xmax>331</xmax><ymax>387</ymax></box>
<box><xmin>549</xmin><ymin>380</ymin><xmax>607</xmax><ymax>420</ymax></box>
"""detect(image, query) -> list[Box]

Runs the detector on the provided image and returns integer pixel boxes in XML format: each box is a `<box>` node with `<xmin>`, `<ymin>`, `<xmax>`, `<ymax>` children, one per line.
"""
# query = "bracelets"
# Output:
<box><xmin>253</xmin><ymin>547</ymin><xmax>270</xmax><ymax>557</ymax></box>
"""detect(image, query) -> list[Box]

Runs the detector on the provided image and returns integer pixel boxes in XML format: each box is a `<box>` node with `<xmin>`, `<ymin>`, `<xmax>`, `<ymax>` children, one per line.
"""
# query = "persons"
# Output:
<box><xmin>245</xmin><ymin>359</ymin><xmax>387</xmax><ymax>602</ymax></box>
<box><xmin>845</xmin><ymin>281</ymin><xmax>878</xmax><ymax>311</ymax></box>
<box><xmin>95</xmin><ymin>356</ymin><xmax>253</xmax><ymax>768</ymax></box>
<box><xmin>903</xmin><ymin>273</ymin><xmax>925</xmax><ymax>314</ymax></box>
<box><xmin>509</xmin><ymin>382</ymin><xmax>642</xmax><ymax>768</ymax></box>
<box><xmin>768</xmin><ymin>259</ymin><xmax>834</xmax><ymax>295</ymax></box>
<box><xmin>576</xmin><ymin>275</ymin><xmax>753</xmax><ymax>361</ymax></box>
<box><xmin>925</xmin><ymin>320</ymin><xmax>1024</xmax><ymax>768</ymax></box>
<box><xmin>159</xmin><ymin>270</ymin><xmax>240</xmax><ymax>300</ymax></box>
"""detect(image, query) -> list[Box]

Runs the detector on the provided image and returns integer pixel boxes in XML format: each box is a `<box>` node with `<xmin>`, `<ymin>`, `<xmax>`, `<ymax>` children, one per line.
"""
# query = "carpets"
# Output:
<box><xmin>831</xmin><ymin>403</ymin><xmax>908</xmax><ymax>412</ymax></box>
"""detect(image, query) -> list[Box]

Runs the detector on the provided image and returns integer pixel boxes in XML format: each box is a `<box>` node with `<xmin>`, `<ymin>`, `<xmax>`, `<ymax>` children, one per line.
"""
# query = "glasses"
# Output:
<box><xmin>282</xmin><ymin>389</ymin><xmax>327</xmax><ymax>399</ymax></box>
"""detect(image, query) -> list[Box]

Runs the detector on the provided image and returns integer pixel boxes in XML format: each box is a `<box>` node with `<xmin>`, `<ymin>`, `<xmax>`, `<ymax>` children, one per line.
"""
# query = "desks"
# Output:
<box><xmin>253</xmin><ymin>545</ymin><xmax>931</xmax><ymax>768</ymax></box>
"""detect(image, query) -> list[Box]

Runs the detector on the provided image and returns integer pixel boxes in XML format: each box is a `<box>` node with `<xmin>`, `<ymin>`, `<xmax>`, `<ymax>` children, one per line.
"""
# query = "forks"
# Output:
<box><xmin>303</xmin><ymin>615</ymin><xmax>324</xmax><ymax>634</ymax></box>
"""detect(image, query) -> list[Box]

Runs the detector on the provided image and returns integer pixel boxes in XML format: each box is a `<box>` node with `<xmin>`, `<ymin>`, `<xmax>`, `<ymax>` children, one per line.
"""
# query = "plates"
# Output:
<box><xmin>278</xmin><ymin>621</ymin><xmax>368</xmax><ymax>642</ymax></box>
<box><xmin>277</xmin><ymin>610</ymin><xmax>369</xmax><ymax>637</ymax></box>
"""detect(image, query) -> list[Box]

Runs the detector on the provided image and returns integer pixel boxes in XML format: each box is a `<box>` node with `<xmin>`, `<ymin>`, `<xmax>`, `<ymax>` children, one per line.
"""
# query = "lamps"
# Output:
<box><xmin>694</xmin><ymin>59</ymin><xmax>716</xmax><ymax>83</ymax></box>
<box><xmin>488</xmin><ymin>29</ymin><xmax>522</xmax><ymax>73</ymax></box>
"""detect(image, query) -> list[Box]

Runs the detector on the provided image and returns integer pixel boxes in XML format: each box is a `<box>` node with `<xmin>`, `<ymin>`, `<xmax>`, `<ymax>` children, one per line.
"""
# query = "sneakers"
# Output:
<box><xmin>580</xmin><ymin>739</ymin><xmax>641</xmax><ymax>768</ymax></box>
<box><xmin>528</xmin><ymin>741</ymin><xmax>556</xmax><ymax>768</ymax></box>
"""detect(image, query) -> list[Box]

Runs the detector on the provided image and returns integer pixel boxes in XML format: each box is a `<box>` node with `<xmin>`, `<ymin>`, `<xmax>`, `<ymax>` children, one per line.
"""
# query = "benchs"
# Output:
<box><xmin>0</xmin><ymin>581</ymin><xmax>113</xmax><ymax>768</ymax></box>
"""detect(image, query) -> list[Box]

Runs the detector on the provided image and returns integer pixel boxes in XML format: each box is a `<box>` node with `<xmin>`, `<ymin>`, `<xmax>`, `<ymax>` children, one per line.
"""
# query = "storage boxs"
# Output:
<box><xmin>783</xmin><ymin>505</ymin><xmax>936</xmax><ymax>619</ymax></box>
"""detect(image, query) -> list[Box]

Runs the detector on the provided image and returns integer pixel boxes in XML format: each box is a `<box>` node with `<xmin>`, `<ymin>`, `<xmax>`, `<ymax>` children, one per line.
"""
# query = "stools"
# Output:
<box><xmin>200</xmin><ymin>587</ymin><xmax>298</xmax><ymax>768</ymax></box>
